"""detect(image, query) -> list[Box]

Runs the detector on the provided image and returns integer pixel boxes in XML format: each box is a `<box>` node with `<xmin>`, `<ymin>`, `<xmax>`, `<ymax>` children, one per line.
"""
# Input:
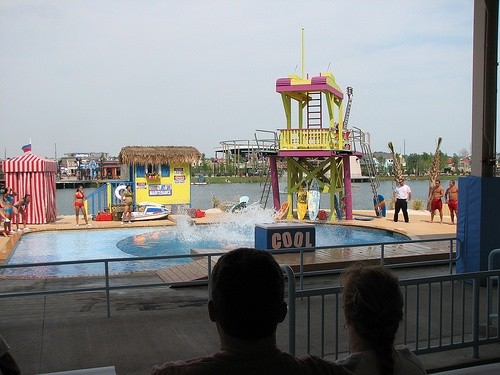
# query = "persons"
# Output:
<box><xmin>73</xmin><ymin>185</ymin><xmax>92</xmax><ymax>226</ymax></box>
<box><xmin>444</xmin><ymin>180</ymin><xmax>458</xmax><ymax>224</ymax></box>
<box><xmin>430</xmin><ymin>179</ymin><xmax>444</xmax><ymax>223</ymax></box>
<box><xmin>0</xmin><ymin>187</ymin><xmax>32</xmax><ymax>237</ymax></box>
<box><xmin>393</xmin><ymin>179</ymin><xmax>412</xmax><ymax>222</ymax></box>
<box><xmin>55</xmin><ymin>171</ymin><xmax>80</xmax><ymax>182</ymax></box>
<box><xmin>122</xmin><ymin>185</ymin><xmax>135</xmax><ymax>224</ymax></box>
<box><xmin>148</xmin><ymin>248</ymin><xmax>426</xmax><ymax>375</ymax></box>
<box><xmin>0</xmin><ymin>335</ymin><xmax>20</xmax><ymax>375</ymax></box>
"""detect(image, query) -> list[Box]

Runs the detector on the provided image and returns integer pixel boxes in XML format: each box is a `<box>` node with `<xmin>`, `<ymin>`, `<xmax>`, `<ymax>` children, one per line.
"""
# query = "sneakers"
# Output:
<box><xmin>23</xmin><ymin>227</ymin><xmax>28</xmax><ymax>230</ymax></box>
<box><xmin>16</xmin><ymin>228</ymin><xmax>21</xmax><ymax>231</ymax></box>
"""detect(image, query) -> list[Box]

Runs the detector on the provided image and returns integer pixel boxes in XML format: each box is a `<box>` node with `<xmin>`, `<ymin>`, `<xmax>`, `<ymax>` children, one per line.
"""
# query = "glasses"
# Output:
<box><xmin>399</xmin><ymin>181</ymin><xmax>403</xmax><ymax>183</ymax></box>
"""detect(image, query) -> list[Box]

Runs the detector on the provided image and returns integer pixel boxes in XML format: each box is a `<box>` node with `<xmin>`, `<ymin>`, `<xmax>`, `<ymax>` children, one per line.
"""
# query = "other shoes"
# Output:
<box><xmin>121</xmin><ymin>222</ymin><xmax>124</xmax><ymax>225</ymax></box>
<box><xmin>76</xmin><ymin>224</ymin><xmax>79</xmax><ymax>227</ymax></box>
<box><xmin>85</xmin><ymin>224</ymin><xmax>91</xmax><ymax>226</ymax></box>
<box><xmin>128</xmin><ymin>221</ymin><xmax>131</xmax><ymax>223</ymax></box>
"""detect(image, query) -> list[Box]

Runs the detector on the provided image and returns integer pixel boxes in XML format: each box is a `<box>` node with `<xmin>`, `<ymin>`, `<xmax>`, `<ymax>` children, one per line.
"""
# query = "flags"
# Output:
<box><xmin>22</xmin><ymin>144</ymin><xmax>31</xmax><ymax>153</ymax></box>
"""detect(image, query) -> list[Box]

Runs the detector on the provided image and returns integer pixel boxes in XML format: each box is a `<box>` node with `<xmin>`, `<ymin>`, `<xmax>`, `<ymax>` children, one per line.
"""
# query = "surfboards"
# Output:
<box><xmin>296</xmin><ymin>178</ymin><xmax>308</xmax><ymax>220</ymax></box>
<box><xmin>308</xmin><ymin>177</ymin><xmax>320</xmax><ymax>221</ymax></box>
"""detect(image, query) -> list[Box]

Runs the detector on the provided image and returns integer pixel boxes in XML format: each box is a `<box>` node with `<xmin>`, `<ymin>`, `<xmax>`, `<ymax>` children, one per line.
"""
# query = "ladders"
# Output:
<box><xmin>259</xmin><ymin>144</ymin><xmax>279</xmax><ymax>210</ymax></box>
<box><xmin>361</xmin><ymin>142</ymin><xmax>382</xmax><ymax>218</ymax></box>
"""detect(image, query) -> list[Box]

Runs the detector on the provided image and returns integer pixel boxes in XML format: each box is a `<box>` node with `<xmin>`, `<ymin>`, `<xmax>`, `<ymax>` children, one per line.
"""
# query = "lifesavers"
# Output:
<box><xmin>275</xmin><ymin>201</ymin><xmax>289</xmax><ymax>220</ymax></box>
